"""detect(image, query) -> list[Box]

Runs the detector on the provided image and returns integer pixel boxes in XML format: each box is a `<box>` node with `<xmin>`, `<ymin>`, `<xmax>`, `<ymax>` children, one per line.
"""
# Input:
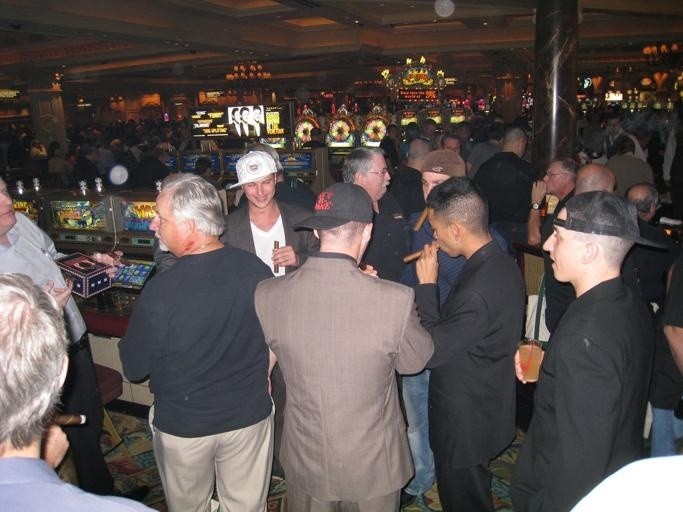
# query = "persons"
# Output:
<box><xmin>117</xmin><ymin>172</ymin><xmax>279</xmax><ymax>511</ymax></box>
<box><xmin>0</xmin><ymin>178</ymin><xmax>123</xmax><ymax>497</ymax></box>
<box><xmin>527</xmin><ymin>158</ymin><xmax>576</xmax><ymax>333</ymax></box>
<box><xmin>396</xmin><ymin>149</ymin><xmax>508</xmax><ymax>511</ymax></box>
<box><xmin>510</xmin><ymin>190</ymin><xmax>666</xmax><ymax>511</ymax></box>
<box><xmin>574</xmin><ymin>99</ymin><xmax>683</xmax><ymax>224</ymax></box>
<box><xmin>229</xmin><ymin>105</ymin><xmax>267</xmax><ymax>137</ymax></box>
<box><xmin>625</xmin><ymin>183</ymin><xmax>675</xmax><ymax>302</ymax></box>
<box><xmin>382</xmin><ymin>118</ymin><xmax>502</xmax><ymax>180</ymax></box>
<box><xmin>649</xmin><ymin>259</ymin><xmax>682</xmax><ymax>458</ymax></box>
<box><xmin>388</xmin><ymin>137</ymin><xmax>426</xmax><ymax>216</ymax></box>
<box><xmin>218</xmin><ymin>151</ymin><xmax>322</xmax><ymax>478</ymax></box>
<box><xmin>342</xmin><ymin>147</ymin><xmax>411</xmax><ymax>281</ymax></box>
<box><xmin>255</xmin><ymin>183</ymin><xmax>434</xmax><ymax>511</ymax></box>
<box><xmin>7</xmin><ymin>117</ymin><xmax>200</xmax><ymax>190</ymax></box>
<box><xmin>473</xmin><ymin>125</ymin><xmax>539</xmax><ymax>242</ymax></box>
<box><xmin>1</xmin><ymin>273</ymin><xmax>155</xmax><ymax>511</ymax></box>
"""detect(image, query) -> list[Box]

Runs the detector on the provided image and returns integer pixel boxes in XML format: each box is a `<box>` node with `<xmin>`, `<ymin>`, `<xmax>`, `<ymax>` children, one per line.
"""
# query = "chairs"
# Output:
<box><xmin>93</xmin><ymin>363</ymin><xmax>130</xmax><ymax>457</ymax></box>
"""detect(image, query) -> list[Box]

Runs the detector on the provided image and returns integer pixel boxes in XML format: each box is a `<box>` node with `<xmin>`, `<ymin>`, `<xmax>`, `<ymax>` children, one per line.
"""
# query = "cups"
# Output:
<box><xmin>519</xmin><ymin>342</ymin><xmax>542</xmax><ymax>383</ymax></box>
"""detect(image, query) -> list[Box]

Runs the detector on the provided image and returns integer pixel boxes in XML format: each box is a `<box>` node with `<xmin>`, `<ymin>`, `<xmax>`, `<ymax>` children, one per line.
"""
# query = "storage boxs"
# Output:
<box><xmin>54</xmin><ymin>252</ymin><xmax>112</xmax><ymax>298</ymax></box>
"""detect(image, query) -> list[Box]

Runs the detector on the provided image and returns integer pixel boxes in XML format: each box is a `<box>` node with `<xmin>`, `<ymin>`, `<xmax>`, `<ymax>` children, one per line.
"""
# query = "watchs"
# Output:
<box><xmin>529</xmin><ymin>203</ymin><xmax>542</xmax><ymax>210</ymax></box>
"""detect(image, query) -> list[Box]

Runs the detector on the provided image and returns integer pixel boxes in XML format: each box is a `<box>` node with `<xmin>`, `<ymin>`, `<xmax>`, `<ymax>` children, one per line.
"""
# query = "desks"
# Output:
<box><xmin>515</xmin><ymin>247</ymin><xmax>545</xmax><ymax>295</ymax></box>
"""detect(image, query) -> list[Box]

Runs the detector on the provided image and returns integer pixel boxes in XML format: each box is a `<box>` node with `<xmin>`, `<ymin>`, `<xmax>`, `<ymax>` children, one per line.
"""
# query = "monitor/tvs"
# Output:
<box><xmin>47</xmin><ymin>200</ymin><xmax>108</xmax><ymax>232</ymax></box>
<box><xmin>277</xmin><ymin>150</ymin><xmax>316</xmax><ymax>178</ymax></box>
<box><xmin>225</xmin><ymin>104</ymin><xmax>266</xmax><ymax>138</ymax></box>
<box><xmin>180</xmin><ymin>152</ymin><xmax>220</xmax><ymax>180</ymax></box>
<box><xmin>400</xmin><ymin>111</ymin><xmax>417</xmax><ymax>125</ymax></box>
<box><xmin>155</xmin><ymin>152</ymin><xmax>178</xmax><ymax>174</ymax></box>
<box><xmin>450</xmin><ymin>109</ymin><xmax>465</xmax><ymax>125</ymax></box>
<box><xmin>221</xmin><ymin>151</ymin><xmax>243</xmax><ymax>176</ymax></box>
<box><xmin>190</xmin><ymin>111</ymin><xmax>227</xmax><ymax>138</ymax></box>
<box><xmin>11</xmin><ymin>197</ymin><xmax>40</xmax><ymax>226</ymax></box>
<box><xmin>425</xmin><ymin>110</ymin><xmax>441</xmax><ymax>124</ymax></box>
<box><xmin>115</xmin><ymin>200</ymin><xmax>157</xmax><ymax>234</ymax></box>
<box><xmin>264</xmin><ymin>110</ymin><xmax>287</xmax><ymax>134</ymax></box>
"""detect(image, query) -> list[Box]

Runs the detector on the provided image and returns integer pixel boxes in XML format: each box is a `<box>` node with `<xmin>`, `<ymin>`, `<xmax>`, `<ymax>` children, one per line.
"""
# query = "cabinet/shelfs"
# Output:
<box><xmin>86</xmin><ymin>332</ymin><xmax>155</xmax><ymax>409</ymax></box>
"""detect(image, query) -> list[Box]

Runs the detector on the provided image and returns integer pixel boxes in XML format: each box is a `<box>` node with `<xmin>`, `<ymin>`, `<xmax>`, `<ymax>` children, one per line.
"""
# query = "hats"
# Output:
<box><xmin>422</xmin><ymin>148</ymin><xmax>466</xmax><ymax>177</ymax></box>
<box><xmin>229</xmin><ymin>150</ymin><xmax>277</xmax><ymax>189</ymax></box>
<box><xmin>287</xmin><ymin>182</ymin><xmax>374</xmax><ymax>230</ymax></box>
<box><xmin>553</xmin><ymin>190</ymin><xmax>676</xmax><ymax>249</ymax></box>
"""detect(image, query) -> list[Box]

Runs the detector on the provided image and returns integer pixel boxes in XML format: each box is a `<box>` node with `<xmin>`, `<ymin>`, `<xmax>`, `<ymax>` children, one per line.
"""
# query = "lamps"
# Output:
<box><xmin>435</xmin><ymin>0</ymin><xmax>455</xmax><ymax>18</ymax></box>
<box><xmin>220</xmin><ymin>54</ymin><xmax>278</xmax><ymax>96</ymax></box>
